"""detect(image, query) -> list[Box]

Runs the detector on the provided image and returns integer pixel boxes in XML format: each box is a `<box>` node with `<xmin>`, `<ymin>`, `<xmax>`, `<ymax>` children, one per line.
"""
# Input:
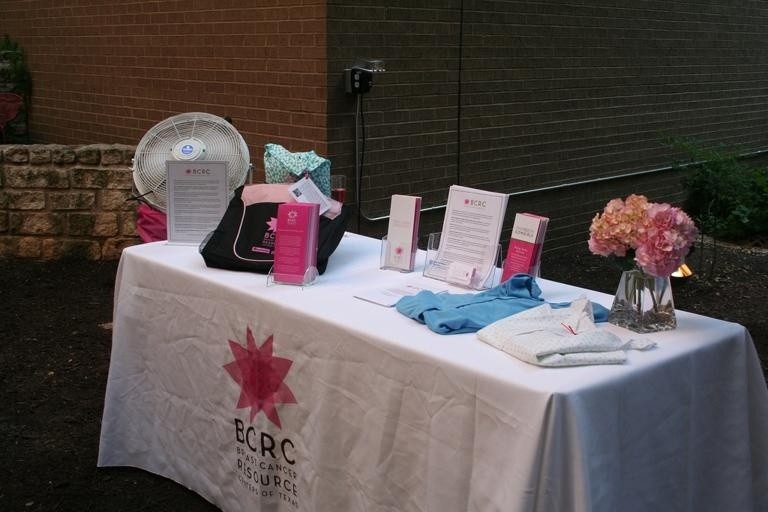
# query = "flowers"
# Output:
<box><xmin>587</xmin><ymin>195</ymin><xmax>697</xmax><ymax>315</ymax></box>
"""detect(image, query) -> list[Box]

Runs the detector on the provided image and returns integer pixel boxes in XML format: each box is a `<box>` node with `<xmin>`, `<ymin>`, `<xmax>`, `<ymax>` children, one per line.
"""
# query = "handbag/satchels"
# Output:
<box><xmin>199</xmin><ymin>179</ymin><xmax>355</xmax><ymax>279</ymax></box>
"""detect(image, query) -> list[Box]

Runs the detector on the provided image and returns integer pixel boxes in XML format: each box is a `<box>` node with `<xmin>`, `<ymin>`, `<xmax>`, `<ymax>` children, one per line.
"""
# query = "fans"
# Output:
<box><xmin>131</xmin><ymin>112</ymin><xmax>251</xmax><ymax>214</ymax></box>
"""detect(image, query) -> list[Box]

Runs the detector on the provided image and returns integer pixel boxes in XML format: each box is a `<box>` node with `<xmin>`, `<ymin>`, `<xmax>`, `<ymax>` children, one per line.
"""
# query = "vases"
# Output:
<box><xmin>608</xmin><ymin>263</ymin><xmax>677</xmax><ymax>334</ymax></box>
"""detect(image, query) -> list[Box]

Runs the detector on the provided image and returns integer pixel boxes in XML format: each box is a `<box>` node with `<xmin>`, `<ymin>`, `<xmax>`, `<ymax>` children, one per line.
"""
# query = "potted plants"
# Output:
<box><xmin>660</xmin><ymin>129</ymin><xmax>768</xmax><ymax>328</ymax></box>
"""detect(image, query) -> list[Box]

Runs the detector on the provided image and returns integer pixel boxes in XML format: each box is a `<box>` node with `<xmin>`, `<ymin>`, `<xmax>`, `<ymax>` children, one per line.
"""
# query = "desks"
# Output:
<box><xmin>125</xmin><ymin>227</ymin><xmax>748</xmax><ymax>512</ymax></box>
